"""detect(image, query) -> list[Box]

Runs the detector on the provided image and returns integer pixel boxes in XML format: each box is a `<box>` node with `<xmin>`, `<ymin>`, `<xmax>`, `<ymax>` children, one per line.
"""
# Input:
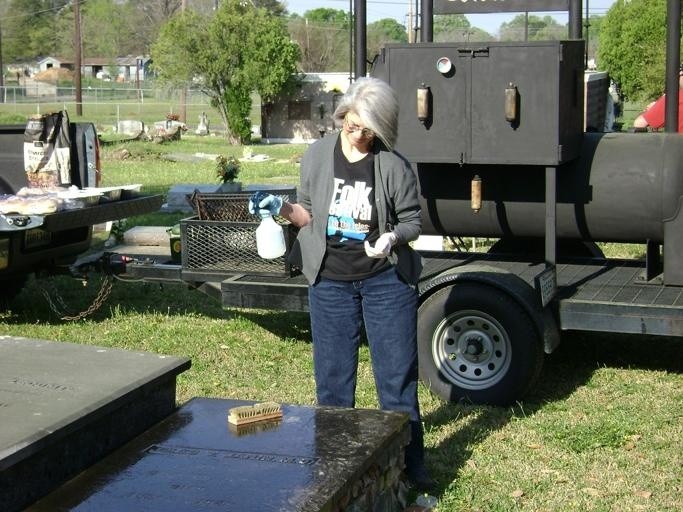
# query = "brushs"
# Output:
<box><xmin>227</xmin><ymin>401</ymin><xmax>285</xmax><ymax>427</ymax></box>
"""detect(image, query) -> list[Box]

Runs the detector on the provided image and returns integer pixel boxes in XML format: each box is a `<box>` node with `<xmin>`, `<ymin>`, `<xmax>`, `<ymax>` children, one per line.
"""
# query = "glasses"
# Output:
<box><xmin>345</xmin><ymin>114</ymin><xmax>375</xmax><ymax>139</ymax></box>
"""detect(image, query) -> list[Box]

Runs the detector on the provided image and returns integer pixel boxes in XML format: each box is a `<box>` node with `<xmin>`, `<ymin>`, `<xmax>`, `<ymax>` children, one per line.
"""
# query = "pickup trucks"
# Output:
<box><xmin>0</xmin><ymin>119</ymin><xmax>166</xmax><ymax>316</ymax></box>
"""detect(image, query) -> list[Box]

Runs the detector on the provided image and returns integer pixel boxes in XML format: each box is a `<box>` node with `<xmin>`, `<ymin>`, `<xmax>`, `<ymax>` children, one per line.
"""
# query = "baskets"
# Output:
<box><xmin>178</xmin><ymin>188</ymin><xmax>300</xmax><ymax>278</ymax></box>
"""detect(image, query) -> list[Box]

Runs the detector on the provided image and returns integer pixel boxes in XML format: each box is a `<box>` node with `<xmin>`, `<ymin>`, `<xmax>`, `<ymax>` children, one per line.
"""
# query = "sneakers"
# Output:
<box><xmin>410</xmin><ymin>462</ymin><xmax>434</xmax><ymax>489</ymax></box>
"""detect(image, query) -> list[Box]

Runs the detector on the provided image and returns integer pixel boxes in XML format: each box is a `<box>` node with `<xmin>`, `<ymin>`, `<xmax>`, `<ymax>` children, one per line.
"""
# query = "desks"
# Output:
<box><xmin>0</xmin><ymin>190</ymin><xmax>167</xmax><ymax>310</ymax></box>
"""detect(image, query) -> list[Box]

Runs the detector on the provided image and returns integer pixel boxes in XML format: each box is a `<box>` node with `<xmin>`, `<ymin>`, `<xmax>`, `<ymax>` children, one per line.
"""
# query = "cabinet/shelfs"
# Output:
<box><xmin>383</xmin><ymin>41</ymin><xmax>585</xmax><ymax>171</ymax></box>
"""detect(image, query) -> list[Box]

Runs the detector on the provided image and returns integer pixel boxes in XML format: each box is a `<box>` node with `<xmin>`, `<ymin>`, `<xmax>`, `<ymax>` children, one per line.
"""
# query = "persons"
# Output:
<box><xmin>248</xmin><ymin>77</ymin><xmax>435</xmax><ymax>487</ymax></box>
<box><xmin>633</xmin><ymin>65</ymin><xmax>683</xmax><ymax>133</ymax></box>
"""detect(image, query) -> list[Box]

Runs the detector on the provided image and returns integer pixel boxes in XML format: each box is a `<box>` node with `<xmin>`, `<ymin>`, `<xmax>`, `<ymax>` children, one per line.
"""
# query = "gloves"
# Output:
<box><xmin>249</xmin><ymin>191</ymin><xmax>283</xmax><ymax>216</ymax></box>
<box><xmin>364</xmin><ymin>232</ymin><xmax>397</xmax><ymax>258</ymax></box>
